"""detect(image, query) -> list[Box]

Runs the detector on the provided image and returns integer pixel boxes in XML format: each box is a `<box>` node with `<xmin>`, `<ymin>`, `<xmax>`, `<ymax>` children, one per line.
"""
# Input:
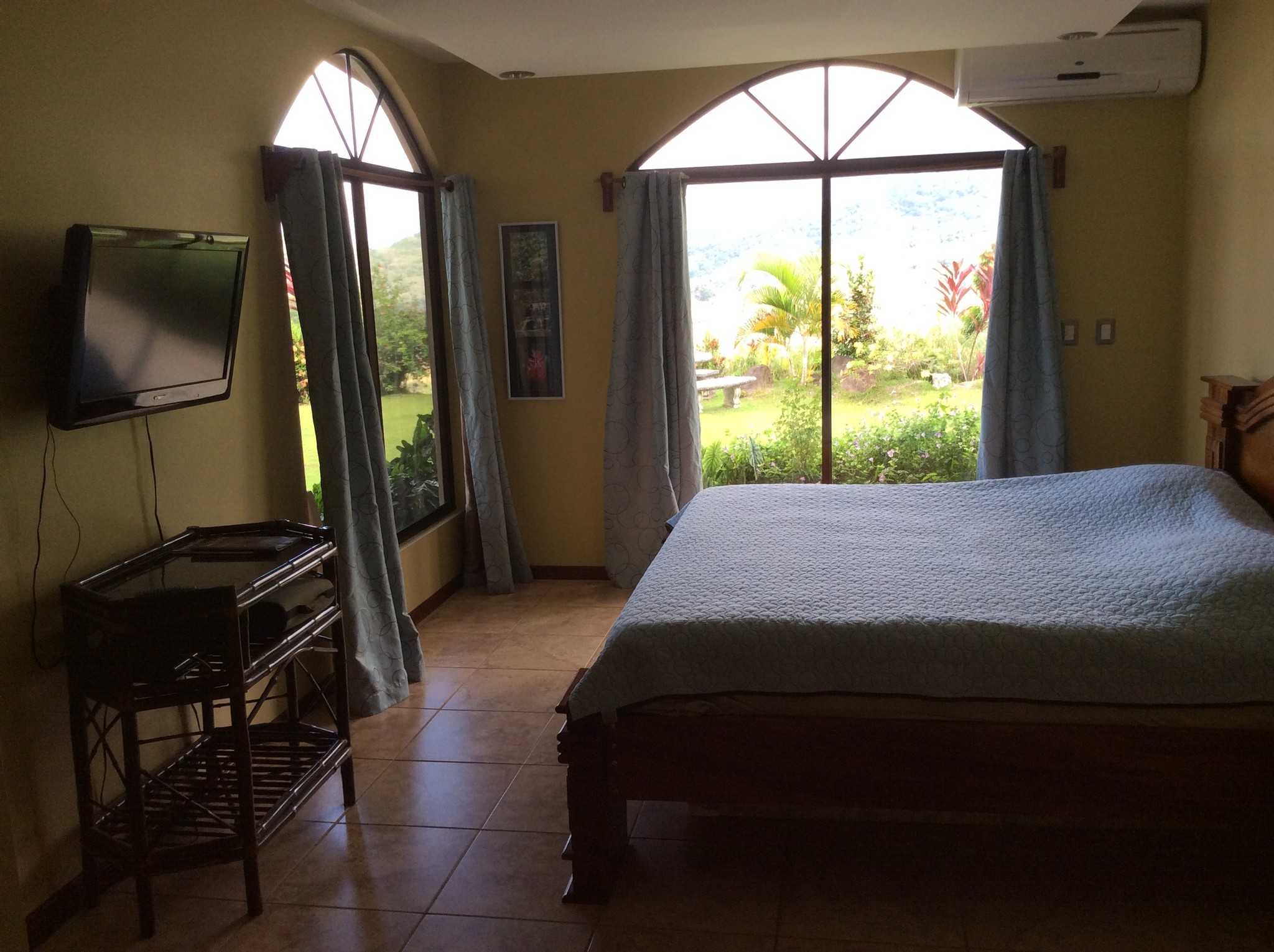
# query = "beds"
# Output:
<box><xmin>555</xmin><ymin>373</ymin><xmax>1273</xmax><ymax>906</ymax></box>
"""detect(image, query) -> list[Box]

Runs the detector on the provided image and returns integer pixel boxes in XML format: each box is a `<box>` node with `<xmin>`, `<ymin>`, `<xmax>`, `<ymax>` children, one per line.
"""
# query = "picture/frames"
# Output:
<box><xmin>498</xmin><ymin>221</ymin><xmax>564</xmax><ymax>400</ymax></box>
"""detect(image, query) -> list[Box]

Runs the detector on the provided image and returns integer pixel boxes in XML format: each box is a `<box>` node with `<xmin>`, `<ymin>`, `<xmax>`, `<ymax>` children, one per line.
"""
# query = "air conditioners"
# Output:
<box><xmin>953</xmin><ymin>20</ymin><xmax>1202</xmax><ymax>108</ymax></box>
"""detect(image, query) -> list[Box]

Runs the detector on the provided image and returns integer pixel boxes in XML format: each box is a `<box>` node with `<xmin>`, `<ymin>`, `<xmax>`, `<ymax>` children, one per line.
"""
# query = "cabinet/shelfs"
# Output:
<box><xmin>60</xmin><ymin>520</ymin><xmax>355</xmax><ymax>940</ymax></box>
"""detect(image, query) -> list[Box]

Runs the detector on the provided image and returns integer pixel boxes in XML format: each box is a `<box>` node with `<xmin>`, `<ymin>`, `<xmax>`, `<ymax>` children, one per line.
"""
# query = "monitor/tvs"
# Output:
<box><xmin>47</xmin><ymin>223</ymin><xmax>252</xmax><ymax>430</ymax></box>
